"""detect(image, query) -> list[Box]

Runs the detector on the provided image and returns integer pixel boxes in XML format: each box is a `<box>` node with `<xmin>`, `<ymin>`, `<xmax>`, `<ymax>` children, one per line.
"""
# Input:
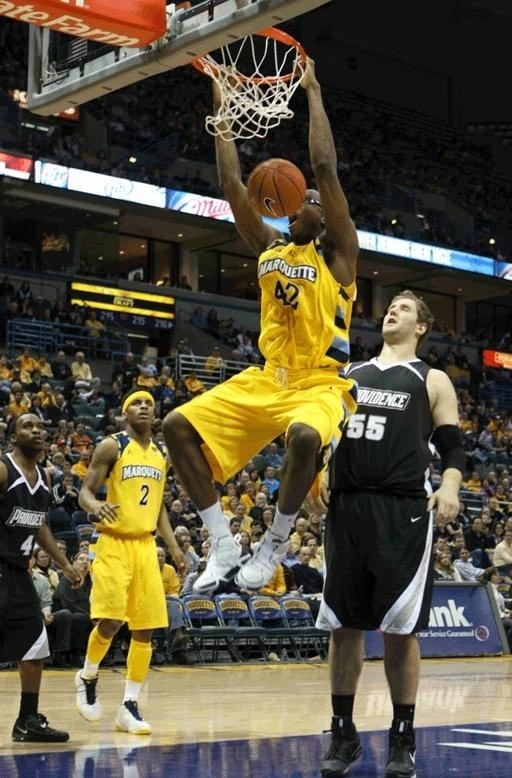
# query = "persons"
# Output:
<box><xmin>2</xmin><ymin>415</ymin><xmax>84</xmax><ymax>741</ymax></box>
<box><xmin>231</xmin><ymin>60</ymin><xmax>319</xmax><ymax>189</ymax></box>
<box><xmin>162</xmin><ymin>54</ymin><xmax>359</xmax><ymax>590</ymax></box>
<box><xmin>74</xmin><ymin>388</ymin><xmax>189</xmax><ymax>735</ymax></box>
<box><xmin>0</xmin><ymin>14</ymin><xmax>511</xmax><ymax>260</ymax></box>
<box><xmin>306</xmin><ymin>285</ymin><xmax>466</xmax><ymax>776</ymax></box>
<box><xmin>258</xmin><ymin>464</ymin><xmax>330</xmax><ymax>624</ymax></box>
<box><xmin>349</xmin><ymin>302</ymin><xmax>510</xmax><ymax>651</ymax></box>
<box><xmin>1</xmin><ymin>248</ymin><xmax>285</xmax><ymax>667</ymax></box>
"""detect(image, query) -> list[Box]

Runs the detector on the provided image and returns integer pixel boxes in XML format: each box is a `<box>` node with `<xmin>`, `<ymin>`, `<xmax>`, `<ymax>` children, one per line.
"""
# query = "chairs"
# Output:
<box><xmin>192</xmin><ymin>532</ymin><xmax>253</xmax><ymax>595</ymax></box>
<box><xmin>235</xmin><ymin>531</ymin><xmax>291</xmax><ymax>591</ymax></box>
<box><xmin>74</xmin><ymin>742</ymin><xmax>101</xmax><ymax>778</ymax></box>
<box><xmin>75</xmin><ymin>670</ymin><xmax>100</xmax><ymax>722</ymax></box>
<box><xmin>384</xmin><ymin>720</ymin><xmax>415</xmax><ymax>777</ymax></box>
<box><xmin>320</xmin><ymin>716</ymin><xmax>363</xmax><ymax>777</ymax></box>
<box><xmin>12</xmin><ymin>716</ymin><xmax>70</xmax><ymax>743</ymax></box>
<box><xmin>114</xmin><ymin>737</ymin><xmax>154</xmax><ymax>767</ymax></box>
<box><xmin>0</xmin><ymin>343</ymin><xmax>512</xmax><ymax>662</ymax></box>
<box><xmin>114</xmin><ymin>702</ymin><xmax>152</xmax><ymax>735</ymax></box>
<box><xmin>12</xmin><ymin>744</ymin><xmax>67</xmax><ymax>771</ymax></box>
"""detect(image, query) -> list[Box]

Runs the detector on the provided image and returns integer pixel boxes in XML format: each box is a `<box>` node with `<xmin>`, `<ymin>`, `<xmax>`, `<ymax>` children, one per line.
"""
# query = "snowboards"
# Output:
<box><xmin>248</xmin><ymin>158</ymin><xmax>306</xmax><ymax>218</ymax></box>
